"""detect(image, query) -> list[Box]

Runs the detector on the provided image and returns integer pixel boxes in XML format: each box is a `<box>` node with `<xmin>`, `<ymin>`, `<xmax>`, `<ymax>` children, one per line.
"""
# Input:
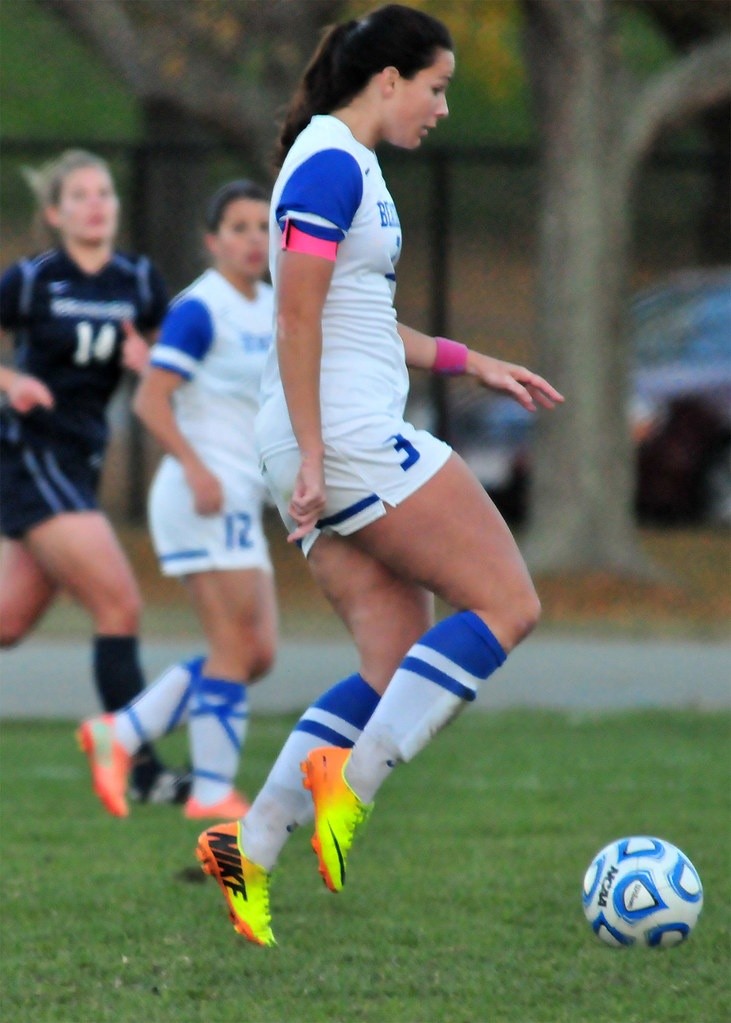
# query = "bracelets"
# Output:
<box><xmin>432</xmin><ymin>337</ymin><xmax>468</xmax><ymax>377</ymax></box>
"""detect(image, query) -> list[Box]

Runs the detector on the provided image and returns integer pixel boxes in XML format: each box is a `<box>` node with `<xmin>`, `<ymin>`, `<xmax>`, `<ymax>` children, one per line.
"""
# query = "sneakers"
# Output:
<box><xmin>299</xmin><ymin>746</ymin><xmax>375</xmax><ymax>895</ymax></box>
<box><xmin>185</xmin><ymin>789</ymin><xmax>250</xmax><ymax>820</ymax></box>
<box><xmin>78</xmin><ymin>713</ymin><xmax>132</xmax><ymax>818</ymax></box>
<box><xmin>194</xmin><ymin>818</ymin><xmax>276</xmax><ymax>947</ymax></box>
<box><xmin>125</xmin><ymin>758</ymin><xmax>194</xmax><ymax>804</ymax></box>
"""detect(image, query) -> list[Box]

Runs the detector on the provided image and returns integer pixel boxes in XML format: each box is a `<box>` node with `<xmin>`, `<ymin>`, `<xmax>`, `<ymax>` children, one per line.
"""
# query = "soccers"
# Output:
<box><xmin>582</xmin><ymin>833</ymin><xmax>705</xmax><ymax>950</ymax></box>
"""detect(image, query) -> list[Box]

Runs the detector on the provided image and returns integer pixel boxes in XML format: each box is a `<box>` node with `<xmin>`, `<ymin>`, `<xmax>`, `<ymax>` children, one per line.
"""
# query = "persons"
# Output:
<box><xmin>0</xmin><ymin>149</ymin><xmax>197</xmax><ymax>806</ymax></box>
<box><xmin>197</xmin><ymin>4</ymin><xmax>567</xmax><ymax>949</ymax></box>
<box><xmin>76</xmin><ymin>186</ymin><xmax>282</xmax><ymax>821</ymax></box>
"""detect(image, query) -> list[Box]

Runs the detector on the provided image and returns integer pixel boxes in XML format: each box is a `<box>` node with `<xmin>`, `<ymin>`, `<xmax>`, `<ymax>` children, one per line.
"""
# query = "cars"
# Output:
<box><xmin>443</xmin><ymin>264</ymin><xmax>731</xmax><ymax>532</ymax></box>
